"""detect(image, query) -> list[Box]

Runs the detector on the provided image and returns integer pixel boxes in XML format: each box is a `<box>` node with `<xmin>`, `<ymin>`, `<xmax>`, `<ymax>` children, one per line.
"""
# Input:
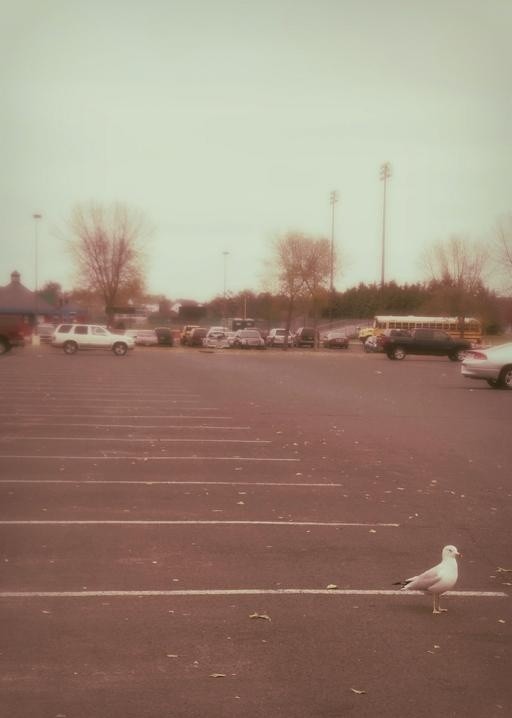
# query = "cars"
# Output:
<box><xmin>322</xmin><ymin>332</ymin><xmax>349</xmax><ymax>349</ymax></box>
<box><xmin>123</xmin><ymin>318</ymin><xmax>267</xmax><ymax>351</ymax></box>
<box><xmin>460</xmin><ymin>342</ymin><xmax>511</xmax><ymax>388</ymax></box>
<box><xmin>36</xmin><ymin>322</ymin><xmax>56</xmax><ymax>342</ymax></box>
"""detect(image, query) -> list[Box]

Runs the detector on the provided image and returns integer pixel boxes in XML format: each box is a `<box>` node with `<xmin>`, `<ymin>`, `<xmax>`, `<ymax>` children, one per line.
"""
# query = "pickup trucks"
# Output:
<box><xmin>376</xmin><ymin>327</ymin><xmax>471</xmax><ymax>362</ymax></box>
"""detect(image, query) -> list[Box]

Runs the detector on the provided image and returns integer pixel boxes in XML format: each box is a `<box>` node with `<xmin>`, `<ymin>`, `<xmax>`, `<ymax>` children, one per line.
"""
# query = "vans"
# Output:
<box><xmin>293</xmin><ymin>326</ymin><xmax>320</xmax><ymax>348</ymax></box>
<box><xmin>266</xmin><ymin>328</ymin><xmax>294</xmax><ymax>347</ymax></box>
<box><xmin>50</xmin><ymin>321</ymin><xmax>136</xmax><ymax>355</ymax></box>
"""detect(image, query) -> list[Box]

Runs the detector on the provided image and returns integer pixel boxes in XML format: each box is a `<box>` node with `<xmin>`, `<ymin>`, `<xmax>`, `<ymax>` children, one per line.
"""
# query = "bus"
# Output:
<box><xmin>372</xmin><ymin>315</ymin><xmax>483</xmax><ymax>338</ymax></box>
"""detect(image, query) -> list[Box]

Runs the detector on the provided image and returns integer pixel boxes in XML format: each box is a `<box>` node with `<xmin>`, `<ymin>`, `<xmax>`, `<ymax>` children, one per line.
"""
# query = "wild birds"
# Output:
<box><xmin>391</xmin><ymin>544</ymin><xmax>463</xmax><ymax>614</ymax></box>
